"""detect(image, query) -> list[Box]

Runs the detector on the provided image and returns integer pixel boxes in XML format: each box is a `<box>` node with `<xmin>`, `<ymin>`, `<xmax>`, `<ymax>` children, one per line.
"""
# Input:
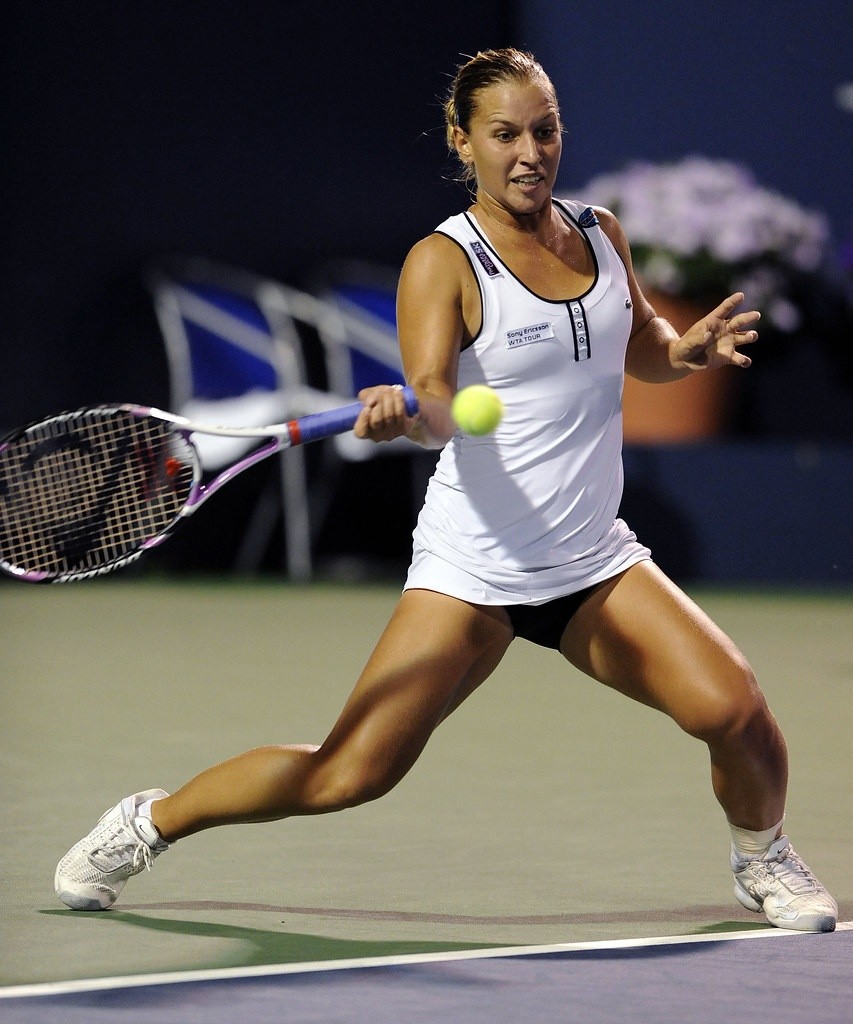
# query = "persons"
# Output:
<box><xmin>53</xmin><ymin>50</ymin><xmax>838</xmax><ymax>933</ymax></box>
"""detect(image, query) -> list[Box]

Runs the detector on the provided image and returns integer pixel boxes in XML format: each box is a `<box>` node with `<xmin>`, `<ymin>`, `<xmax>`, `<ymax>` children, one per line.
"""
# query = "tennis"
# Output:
<box><xmin>452</xmin><ymin>385</ymin><xmax>502</xmax><ymax>435</ymax></box>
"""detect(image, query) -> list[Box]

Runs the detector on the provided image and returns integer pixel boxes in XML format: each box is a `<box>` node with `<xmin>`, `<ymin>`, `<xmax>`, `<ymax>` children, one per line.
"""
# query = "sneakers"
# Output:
<box><xmin>728</xmin><ymin>834</ymin><xmax>839</xmax><ymax>933</ymax></box>
<box><xmin>51</xmin><ymin>787</ymin><xmax>171</xmax><ymax>912</ymax></box>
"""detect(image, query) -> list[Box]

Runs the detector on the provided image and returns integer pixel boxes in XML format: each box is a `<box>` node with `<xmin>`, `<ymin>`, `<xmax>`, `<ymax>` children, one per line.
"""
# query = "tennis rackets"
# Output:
<box><xmin>0</xmin><ymin>386</ymin><xmax>419</xmax><ymax>583</ymax></box>
